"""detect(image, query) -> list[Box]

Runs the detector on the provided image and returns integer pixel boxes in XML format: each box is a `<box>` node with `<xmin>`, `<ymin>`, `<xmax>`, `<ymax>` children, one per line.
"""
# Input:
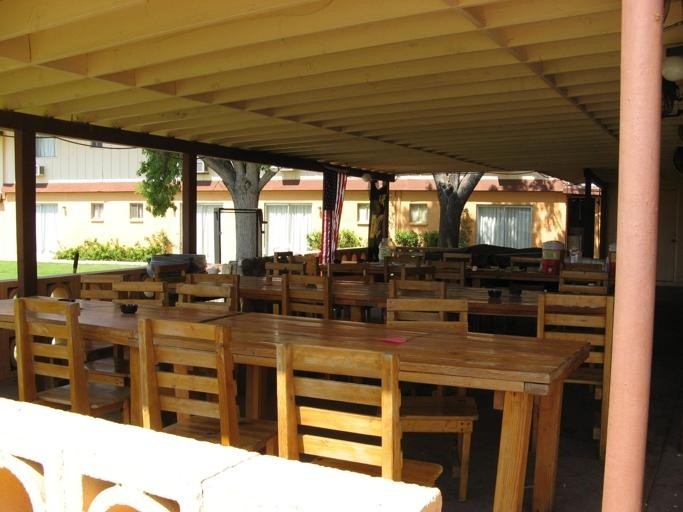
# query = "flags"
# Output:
<box><xmin>318</xmin><ymin>171</ymin><xmax>346</xmax><ymax>277</ymax></box>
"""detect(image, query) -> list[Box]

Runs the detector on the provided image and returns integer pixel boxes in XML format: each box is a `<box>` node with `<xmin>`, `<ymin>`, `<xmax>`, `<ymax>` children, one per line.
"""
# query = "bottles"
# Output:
<box><xmin>377</xmin><ymin>238</ymin><xmax>397</xmax><ymax>263</ymax></box>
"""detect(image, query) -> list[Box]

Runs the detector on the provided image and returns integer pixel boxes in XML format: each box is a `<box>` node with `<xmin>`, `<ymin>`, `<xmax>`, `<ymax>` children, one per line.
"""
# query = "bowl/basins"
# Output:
<box><xmin>120</xmin><ymin>304</ymin><xmax>138</xmax><ymax>314</ymax></box>
